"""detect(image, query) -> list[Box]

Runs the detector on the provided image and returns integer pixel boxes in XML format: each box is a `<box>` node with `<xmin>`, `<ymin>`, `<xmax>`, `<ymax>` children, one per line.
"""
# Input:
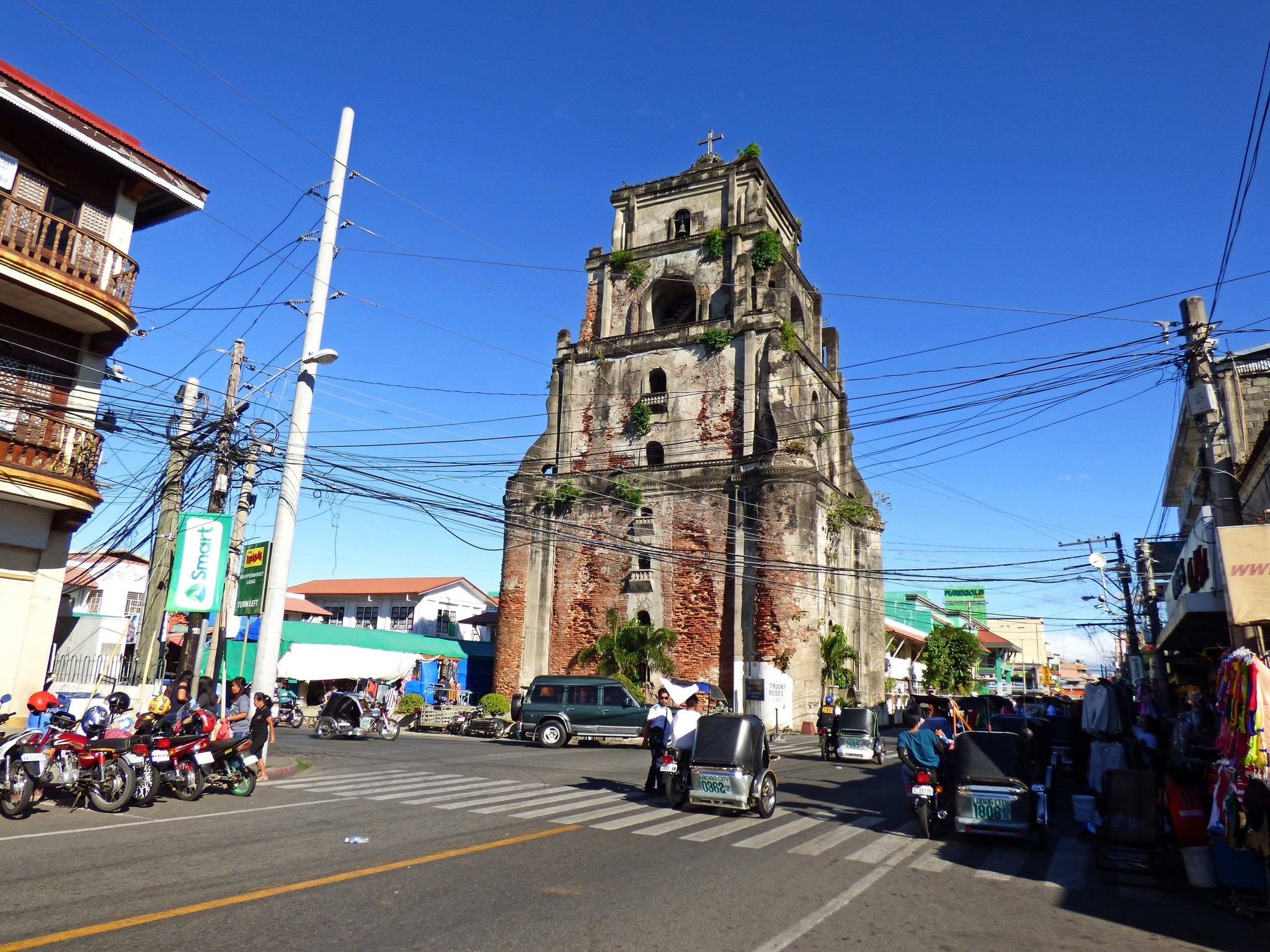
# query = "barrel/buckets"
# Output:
<box><xmin>1180</xmin><ymin>847</ymin><xmax>1217</xmax><ymax>890</ymax></box>
<box><xmin>1072</xmin><ymin>795</ymin><xmax>1096</xmax><ymax>822</ymax></box>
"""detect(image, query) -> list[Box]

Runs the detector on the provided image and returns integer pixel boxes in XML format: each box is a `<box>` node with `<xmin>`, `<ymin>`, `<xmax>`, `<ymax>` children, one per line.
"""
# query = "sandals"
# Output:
<box><xmin>256</xmin><ymin>775</ymin><xmax>269</xmax><ymax>782</ymax></box>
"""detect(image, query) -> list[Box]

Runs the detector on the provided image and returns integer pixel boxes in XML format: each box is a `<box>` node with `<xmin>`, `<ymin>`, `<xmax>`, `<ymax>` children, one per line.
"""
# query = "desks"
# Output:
<box><xmin>295</xmin><ymin>715</ymin><xmax>318</xmax><ymax>727</ymax></box>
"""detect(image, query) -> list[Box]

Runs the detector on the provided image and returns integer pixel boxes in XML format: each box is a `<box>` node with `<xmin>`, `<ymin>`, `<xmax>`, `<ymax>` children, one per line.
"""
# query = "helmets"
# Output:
<box><xmin>193</xmin><ymin>709</ymin><xmax>216</xmax><ymax>734</ymax></box>
<box><xmin>148</xmin><ymin>695</ymin><xmax>172</xmax><ymax>715</ymax></box>
<box><xmin>27</xmin><ymin>691</ymin><xmax>56</xmax><ymax>715</ymax></box>
<box><xmin>81</xmin><ymin>706</ymin><xmax>108</xmax><ymax>736</ymax></box>
<box><xmin>108</xmin><ymin>691</ymin><xmax>131</xmax><ymax>713</ymax></box>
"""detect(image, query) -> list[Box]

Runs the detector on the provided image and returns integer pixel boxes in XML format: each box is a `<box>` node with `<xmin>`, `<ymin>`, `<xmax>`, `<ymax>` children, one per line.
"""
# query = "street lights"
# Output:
<box><xmin>171</xmin><ymin>337</ymin><xmax>341</xmax><ymax>679</ymax></box>
<box><xmin>1082</xmin><ymin>595</ymin><xmax>1140</xmax><ymax>653</ymax></box>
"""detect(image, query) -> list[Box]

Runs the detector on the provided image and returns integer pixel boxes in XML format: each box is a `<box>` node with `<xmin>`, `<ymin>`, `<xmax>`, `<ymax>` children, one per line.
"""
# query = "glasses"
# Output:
<box><xmin>659</xmin><ymin>696</ymin><xmax>670</xmax><ymax>700</ymax></box>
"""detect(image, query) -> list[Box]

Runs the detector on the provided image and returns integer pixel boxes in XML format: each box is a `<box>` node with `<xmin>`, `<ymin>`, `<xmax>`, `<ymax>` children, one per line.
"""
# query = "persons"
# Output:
<box><xmin>437</xmin><ymin>676</ymin><xmax>451</xmax><ymax>704</ymax></box>
<box><xmin>641</xmin><ymin>688</ymin><xmax>673</xmax><ymax>794</ymax></box>
<box><xmin>896</xmin><ymin>677</ymin><xmax>1056</xmax><ymax>816</ymax></box>
<box><xmin>162</xmin><ymin>670</ymin><xmax>275</xmax><ymax>781</ymax></box>
<box><xmin>324</xmin><ymin>686</ymin><xmax>338</xmax><ymax>702</ymax></box>
<box><xmin>359</xmin><ymin>677</ymin><xmax>399</xmax><ymax>718</ymax></box>
<box><xmin>671</xmin><ymin>694</ymin><xmax>702</xmax><ymax>750</ymax></box>
<box><xmin>708</xmin><ymin>699</ymin><xmax>728</xmax><ymax>716</ymax></box>
<box><xmin>816</xmin><ymin>696</ymin><xmax>842</xmax><ymax>731</ymax></box>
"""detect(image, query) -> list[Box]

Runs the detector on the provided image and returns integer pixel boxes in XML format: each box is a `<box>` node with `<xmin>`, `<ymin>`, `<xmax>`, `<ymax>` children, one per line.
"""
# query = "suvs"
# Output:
<box><xmin>510</xmin><ymin>675</ymin><xmax>683</xmax><ymax>749</ymax></box>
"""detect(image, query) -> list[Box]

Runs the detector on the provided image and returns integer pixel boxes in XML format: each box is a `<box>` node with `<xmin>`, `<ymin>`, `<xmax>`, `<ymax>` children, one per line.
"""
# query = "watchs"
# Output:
<box><xmin>271</xmin><ymin>738</ymin><xmax>274</xmax><ymax>740</ymax></box>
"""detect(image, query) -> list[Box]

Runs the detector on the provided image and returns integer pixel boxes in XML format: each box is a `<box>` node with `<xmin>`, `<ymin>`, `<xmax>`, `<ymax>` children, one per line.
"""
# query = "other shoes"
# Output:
<box><xmin>659</xmin><ymin>790</ymin><xmax>666</xmax><ymax>794</ymax></box>
<box><xmin>909</xmin><ymin>800</ymin><xmax>915</xmax><ymax>807</ymax></box>
<box><xmin>836</xmin><ymin>756</ymin><xmax>841</xmax><ymax>758</ymax></box>
<box><xmin>645</xmin><ymin>787</ymin><xmax>659</xmax><ymax>793</ymax></box>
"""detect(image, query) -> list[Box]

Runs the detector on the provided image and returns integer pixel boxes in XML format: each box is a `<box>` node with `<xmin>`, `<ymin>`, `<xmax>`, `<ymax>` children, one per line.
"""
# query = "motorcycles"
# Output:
<box><xmin>0</xmin><ymin>695</ymin><xmax>262</xmax><ymax>817</ymax></box>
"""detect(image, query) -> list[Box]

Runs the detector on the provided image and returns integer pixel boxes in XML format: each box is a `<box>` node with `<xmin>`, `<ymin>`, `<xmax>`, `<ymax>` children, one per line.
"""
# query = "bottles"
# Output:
<box><xmin>344</xmin><ymin>837</ymin><xmax>369</xmax><ymax>844</ymax></box>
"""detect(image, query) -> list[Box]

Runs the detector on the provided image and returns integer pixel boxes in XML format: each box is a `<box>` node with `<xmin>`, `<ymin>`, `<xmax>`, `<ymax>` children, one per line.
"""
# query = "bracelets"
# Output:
<box><xmin>227</xmin><ymin>716</ymin><xmax>229</xmax><ymax>721</ymax></box>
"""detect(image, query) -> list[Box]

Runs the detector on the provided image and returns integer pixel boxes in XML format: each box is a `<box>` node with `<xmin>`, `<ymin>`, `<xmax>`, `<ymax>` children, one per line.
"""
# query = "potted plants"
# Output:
<box><xmin>397</xmin><ymin>694</ymin><xmax>426</xmax><ymax>716</ymax></box>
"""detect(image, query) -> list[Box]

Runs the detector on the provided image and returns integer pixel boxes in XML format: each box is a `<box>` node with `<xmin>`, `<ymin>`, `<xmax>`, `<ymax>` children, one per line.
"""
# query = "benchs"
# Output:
<box><xmin>471</xmin><ymin>719</ymin><xmax>494</xmax><ymax>730</ymax></box>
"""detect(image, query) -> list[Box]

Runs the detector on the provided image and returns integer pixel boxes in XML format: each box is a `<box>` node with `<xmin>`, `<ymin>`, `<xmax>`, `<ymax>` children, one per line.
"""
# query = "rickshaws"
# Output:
<box><xmin>449</xmin><ymin>702</ymin><xmax>506</xmax><ymax>739</ymax></box>
<box><xmin>907</xmin><ymin>690</ymin><xmax>1087</xmax><ymax>852</ymax></box>
<box><xmin>818</xmin><ymin>706</ymin><xmax>887</xmax><ymax>763</ymax></box>
<box><xmin>271</xmin><ymin>693</ymin><xmax>305</xmax><ymax>729</ymax></box>
<box><xmin>657</xmin><ymin>713</ymin><xmax>780</xmax><ymax>819</ymax></box>
<box><xmin>314</xmin><ymin>691</ymin><xmax>401</xmax><ymax>740</ymax></box>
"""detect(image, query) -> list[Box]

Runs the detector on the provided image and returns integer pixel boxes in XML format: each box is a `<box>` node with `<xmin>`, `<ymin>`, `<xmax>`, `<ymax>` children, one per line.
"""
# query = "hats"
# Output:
<box><xmin>826</xmin><ymin>696</ymin><xmax>833</xmax><ymax>704</ymax></box>
<box><xmin>927</xmin><ymin>685</ymin><xmax>936</xmax><ymax>692</ymax></box>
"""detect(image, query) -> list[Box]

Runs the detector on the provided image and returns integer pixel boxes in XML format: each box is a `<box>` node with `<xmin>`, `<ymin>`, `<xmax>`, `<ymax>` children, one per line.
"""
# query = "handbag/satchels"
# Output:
<box><xmin>649</xmin><ymin>726</ymin><xmax>665</xmax><ymax>749</ymax></box>
<box><xmin>210</xmin><ymin>717</ymin><xmax>234</xmax><ymax>741</ymax></box>
